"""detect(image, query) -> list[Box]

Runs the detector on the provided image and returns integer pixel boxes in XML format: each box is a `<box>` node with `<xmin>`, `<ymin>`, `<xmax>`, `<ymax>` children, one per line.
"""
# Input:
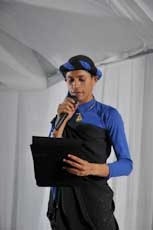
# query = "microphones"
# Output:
<box><xmin>55</xmin><ymin>95</ymin><xmax>77</xmax><ymax>129</ymax></box>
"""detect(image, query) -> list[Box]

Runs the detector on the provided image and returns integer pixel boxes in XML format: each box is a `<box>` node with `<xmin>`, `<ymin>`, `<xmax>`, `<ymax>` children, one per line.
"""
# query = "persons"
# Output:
<box><xmin>46</xmin><ymin>55</ymin><xmax>133</xmax><ymax>230</ymax></box>
